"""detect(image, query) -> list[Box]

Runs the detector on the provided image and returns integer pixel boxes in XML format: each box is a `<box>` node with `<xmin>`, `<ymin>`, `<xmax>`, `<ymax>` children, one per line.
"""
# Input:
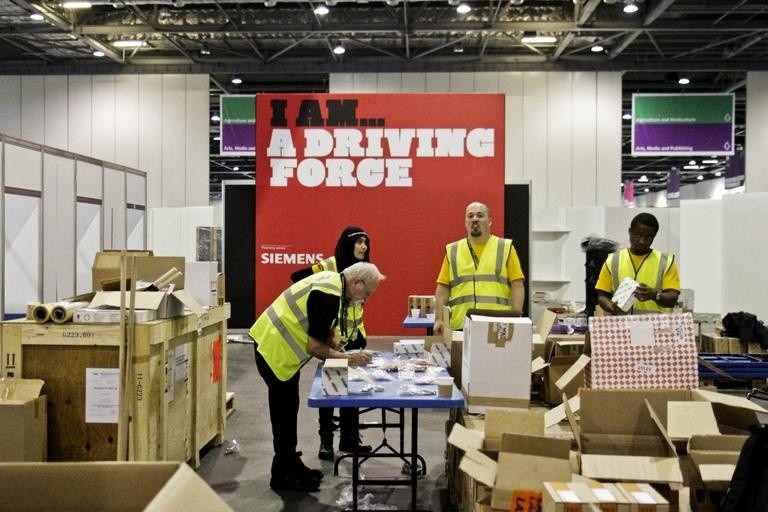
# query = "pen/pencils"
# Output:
<box><xmin>359</xmin><ymin>346</ymin><xmax>363</xmax><ymax>356</ymax></box>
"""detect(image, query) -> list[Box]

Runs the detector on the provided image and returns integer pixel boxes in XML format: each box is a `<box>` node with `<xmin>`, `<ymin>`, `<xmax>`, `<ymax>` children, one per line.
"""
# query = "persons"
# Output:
<box><xmin>433</xmin><ymin>202</ymin><xmax>524</xmax><ymax>336</ymax></box>
<box><xmin>291</xmin><ymin>226</ymin><xmax>372</xmax><ymax>460</ymax></box>
<box><xmin>248</xmin><ymin>262</ymin><xmax>386</xmax><ymax>493</ymax></box>
<box><xmin>594</xmin><ymin>212</ymin><xmax>681</xmax><ymax>315</ymax></box>
<box><xmin>718</xmin><ymin>424</ymin><xmax>768</xmax><ymax>512</ymax></box>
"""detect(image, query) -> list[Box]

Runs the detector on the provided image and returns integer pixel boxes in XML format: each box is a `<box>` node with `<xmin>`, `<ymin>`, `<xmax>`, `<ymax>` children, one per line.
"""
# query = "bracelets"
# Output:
<box><xmin>656</xmin><ymin>291</ymin><xmax>661</xmax><ymax>302</ymax></box>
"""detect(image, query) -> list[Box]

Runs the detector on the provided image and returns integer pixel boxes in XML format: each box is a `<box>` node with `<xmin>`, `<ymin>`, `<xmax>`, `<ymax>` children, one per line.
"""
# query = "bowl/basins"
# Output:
<box><xmin>426</xmin><ymin>314</ymin><xmax>435</xmax><ymax>320</ymax></box>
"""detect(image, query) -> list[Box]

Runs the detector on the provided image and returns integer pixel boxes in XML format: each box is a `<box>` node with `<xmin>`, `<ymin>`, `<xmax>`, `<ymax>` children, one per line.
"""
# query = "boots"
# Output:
<box><xmin>269</xmin><ymin>450</ymin><xmax>324</xmax><ymax>493</ymax></box>
<box><xmin>318</xmin><ymin>433</ymin><xmax>334</xmax><ymax>461</ymax></box>
<box><xmin>338</xmin><ymin>431</ymin><xmax>373</xmax><ymax>454</ymax></box>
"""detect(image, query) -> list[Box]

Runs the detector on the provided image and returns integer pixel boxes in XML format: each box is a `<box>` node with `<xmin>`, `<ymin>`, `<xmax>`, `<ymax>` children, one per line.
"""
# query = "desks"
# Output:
<box><xmin>307</xmin><ymin>353</ymin><xmax>465</xmax><ymax>512</ymax></box>
<box><xmin>0</xmin><ymin>302</ymin><xmax>231</xmax><ymax>467</ymax></box>
<box><xmin>402</xmin><ymin>314</ymin><xmax>434</xmax><ymax>336</ymax></box>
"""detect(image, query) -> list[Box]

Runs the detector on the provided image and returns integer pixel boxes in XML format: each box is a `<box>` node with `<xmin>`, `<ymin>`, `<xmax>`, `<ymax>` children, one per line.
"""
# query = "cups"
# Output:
<box><xmin>411</xmin><ymin>309</ymin><xmax>420</xmax><ymax>321</ymax></box>
<box><xmin>435</xmin><ymin>376</ymin><xmax>453</xmax><ymax>397</ymax></box>
<box><xmin>400</xmin><ymin>360</ymin><xmax>416</xmax><ymax>380</ymax></box>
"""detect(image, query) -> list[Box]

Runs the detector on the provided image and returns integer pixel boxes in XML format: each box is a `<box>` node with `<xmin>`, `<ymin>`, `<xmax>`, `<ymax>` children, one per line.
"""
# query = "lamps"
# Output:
<box><xmin>334</xmin><ymin>40</ymin><xmax>346</xmax><ymax>54</ymax></box>
<box><xmin>623</xmin><ymin>0</ymin><xmax>638</xmax><ymax>13</ymax></box>
<box><xmin>454</xmin><ymin>42</ymin><xmax>463</xmax><ymax>52</ymax></box>
<box><xmin>211</xmin><ymin>111</ymin><xmax>220</xmax><ymax>122</ymax></box>
<box><xmin>230</xmin><ymin>76</ymin><xmax>242</xmax><ymax>84</ymax></box>
<box><xmin>591</xmin><ymin>38</ymin><xmax>603</xmax><ymax>52</ymax></box>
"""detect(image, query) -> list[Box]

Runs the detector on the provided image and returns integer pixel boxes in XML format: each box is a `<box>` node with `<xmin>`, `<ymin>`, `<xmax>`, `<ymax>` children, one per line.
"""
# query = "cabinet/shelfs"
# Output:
<box><xmin>533</xmin><ymin>230</ymin><xmax>570</xmax><ymax>283</ymax></box>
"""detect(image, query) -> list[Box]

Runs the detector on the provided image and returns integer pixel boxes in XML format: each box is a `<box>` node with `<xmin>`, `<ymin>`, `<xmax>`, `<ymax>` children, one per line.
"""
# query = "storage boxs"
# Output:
<box><xmin>93</xmin><ymin>250</ymin><xmax>153</xmax><ymax>292</ymax></box>
<box><xmin>131</xmin><ymin>255</ymin><xmax>185</xmax><ymax>291</ymax></box>
<box><xmin>89</xmin><ymin>292</ymin><xmax>208</xmax><ymax>318</ymax></box>
<box><xmin>408</xmin><ymin>295</ymin><xmax>435</xmax><ymax>317</ymax></box>
<box><xmin>0</xmin><ymin>377</ymin><xmax>47</xmax><ymax>461</ymax></box>
<box><xmin>0</xmin><ymin>460</ymin><xmax>236</xmax><ymax>512</ymax></box>
<box><xmin>185</xmin><ymin>261</ymin><xmax>218</xmax><ymax>305</ymax></box>
